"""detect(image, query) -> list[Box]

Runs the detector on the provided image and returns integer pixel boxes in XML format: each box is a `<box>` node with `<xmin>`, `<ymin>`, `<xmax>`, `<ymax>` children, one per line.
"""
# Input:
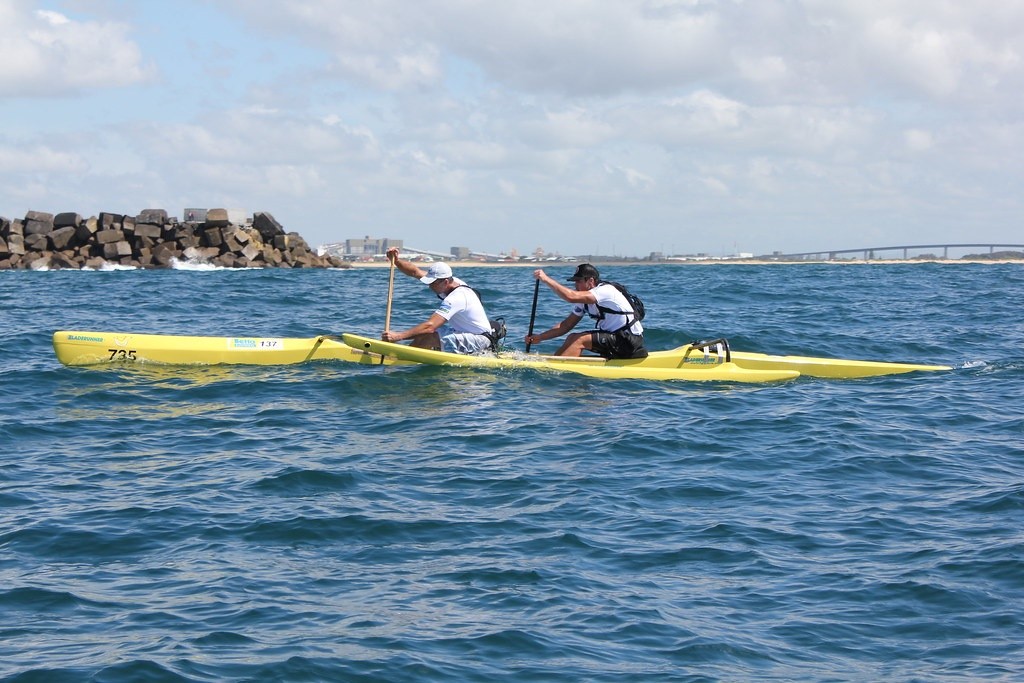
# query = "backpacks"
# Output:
<box><xmin>594</xmin><ymin>281</ymin><xmax>645</xmax><ymax>321</ymax></box>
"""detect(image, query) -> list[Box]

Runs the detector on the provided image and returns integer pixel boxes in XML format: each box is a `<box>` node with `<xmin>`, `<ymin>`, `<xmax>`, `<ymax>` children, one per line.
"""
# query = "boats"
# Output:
<box><xmin>53</xmin><ymin>331</ymin><xmax>954</xmax><ymax>387</ymax></box>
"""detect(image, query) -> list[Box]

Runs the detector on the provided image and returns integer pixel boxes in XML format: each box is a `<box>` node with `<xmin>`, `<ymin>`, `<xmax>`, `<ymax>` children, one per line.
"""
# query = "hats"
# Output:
<box><xmin>419</xmin><ymin>261</ymin><xmax>452</xmax><ymax>284</ymax></box>
<box><xmin>567</xmin><ymin>264</ymin><xmax>598</xmax><ymax>282</ymax></box>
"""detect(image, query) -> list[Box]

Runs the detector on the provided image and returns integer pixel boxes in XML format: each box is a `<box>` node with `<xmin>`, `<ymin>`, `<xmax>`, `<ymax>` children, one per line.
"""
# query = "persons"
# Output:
<box><xmin>525</xmin><ymin>263</ymin><xmax>648</xmax><ymax>359</ymax></box>
<box><xmin>382</xmin><ymin>246</ymin><xmax>491</xmax><ymax>354</ymax></box>
